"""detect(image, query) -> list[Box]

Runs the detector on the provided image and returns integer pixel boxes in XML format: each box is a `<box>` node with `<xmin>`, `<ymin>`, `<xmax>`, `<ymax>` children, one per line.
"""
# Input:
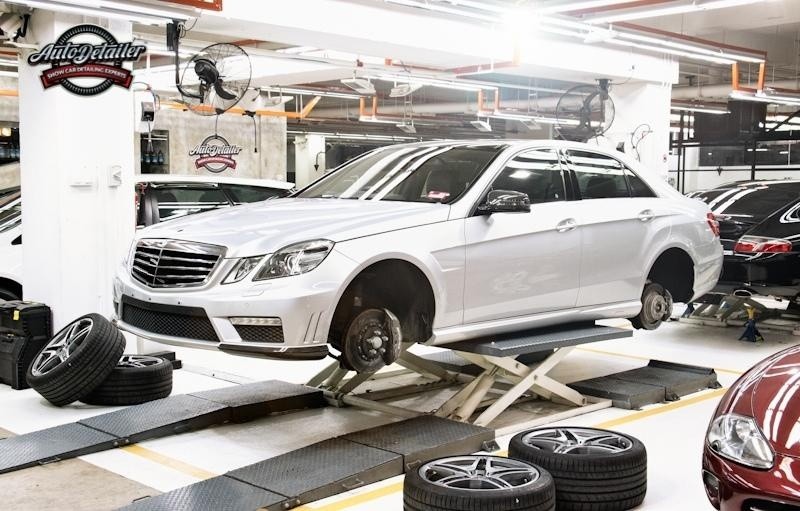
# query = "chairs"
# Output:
<box><xmin>420</xmin><ymin>170</ymin><xmax>617</xmax><ymax>210</ymax></box>
<box><xmin>157</xmin><ymin>191</ymin><xmax>243</xmax><ymax>218</ymax></box>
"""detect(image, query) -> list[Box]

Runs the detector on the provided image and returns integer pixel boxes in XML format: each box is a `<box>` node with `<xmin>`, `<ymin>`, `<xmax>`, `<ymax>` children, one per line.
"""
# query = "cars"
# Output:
<box><xmin>0</xmin><ymin>139</ymin><xmax>800</xmax><ymax>511</ymax></box>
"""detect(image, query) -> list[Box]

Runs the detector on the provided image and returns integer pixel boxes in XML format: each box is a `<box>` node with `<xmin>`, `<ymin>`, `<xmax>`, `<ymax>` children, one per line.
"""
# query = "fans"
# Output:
<box><xmin>166</xmin><ymin>18</ymin><xmax>253</xmax><ymax>116</ymax></box>
<box><xmin>555</xmin><ymin>83</ymin><xmax>615</xmax><ymax>140</ymax></box>
